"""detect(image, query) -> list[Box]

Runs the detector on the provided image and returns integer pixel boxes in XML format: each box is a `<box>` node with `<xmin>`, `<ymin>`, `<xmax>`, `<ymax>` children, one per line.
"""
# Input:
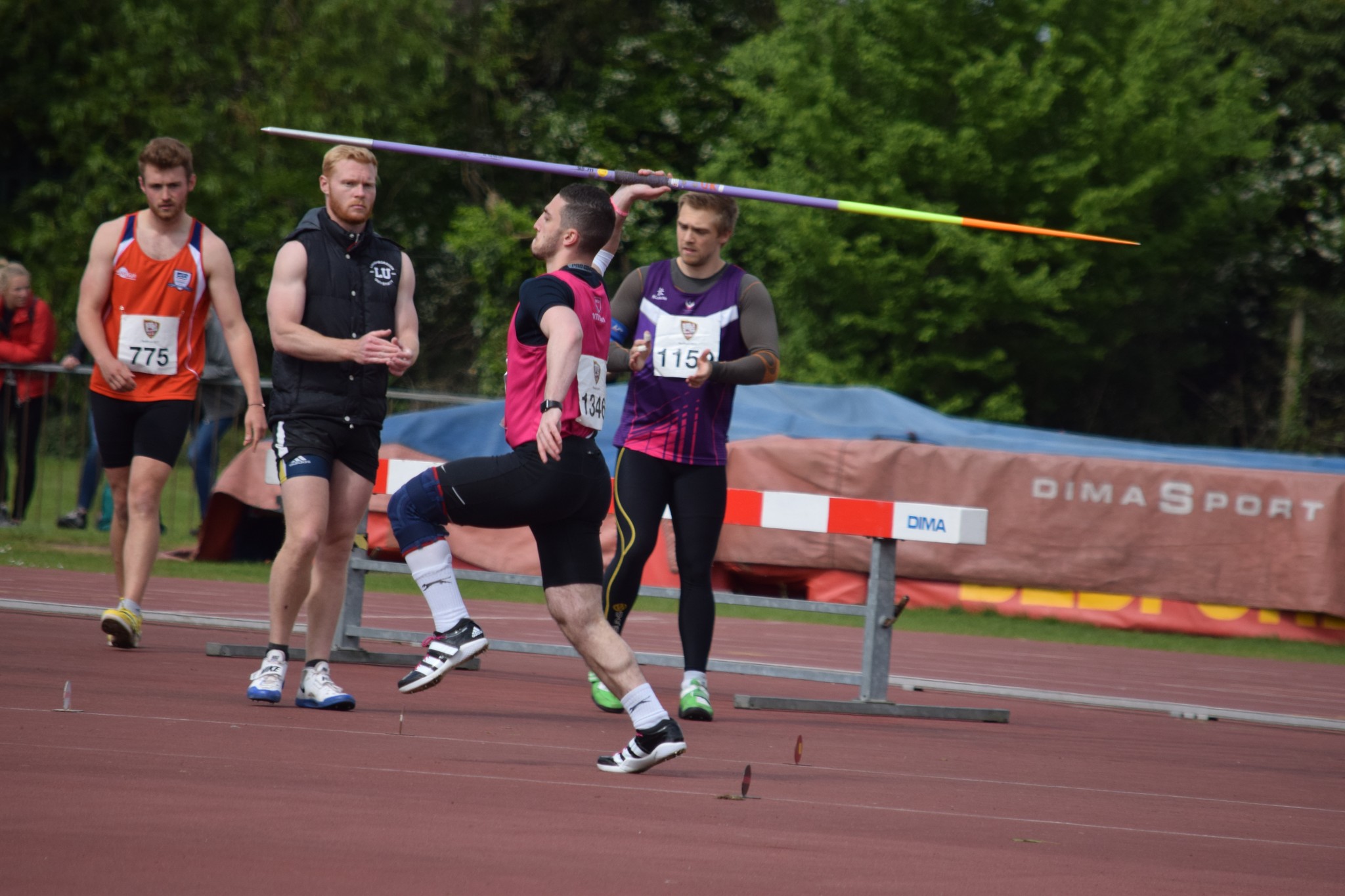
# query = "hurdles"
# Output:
<box><xmin>206</xmin><ymin>445</ymin><xmax>1010</xmax><ymax>726</ymax></box>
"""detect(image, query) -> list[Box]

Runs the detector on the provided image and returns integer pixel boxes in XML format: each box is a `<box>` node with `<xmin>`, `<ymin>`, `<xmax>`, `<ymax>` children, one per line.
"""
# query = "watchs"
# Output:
<box><xmin>540</xmin><ymin>399</ymin><xmax>562</xmax><ymax>412</ymax></box>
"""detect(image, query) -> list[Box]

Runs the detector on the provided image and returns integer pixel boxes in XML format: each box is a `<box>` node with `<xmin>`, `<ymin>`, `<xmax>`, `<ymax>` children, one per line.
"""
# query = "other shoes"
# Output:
<box><xmin>57</xmin><ymin>512</ymin><xmax>87</xmax><ymax>528</ymax></box>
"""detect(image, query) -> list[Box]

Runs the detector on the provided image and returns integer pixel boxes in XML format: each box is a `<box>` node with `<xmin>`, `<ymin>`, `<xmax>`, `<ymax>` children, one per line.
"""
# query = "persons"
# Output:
<box><xmin>73</xmin><ymin>137</ymin><xmax>270</xmax><ymax>645</ymax></box>
<box><xmin>585</xmin><ymin>188</ymin><xmax>781</xmax><ymax>723</ymax></box>
<box><xmin>388</xmin><ymin>167</ymin><xmax>688</xmax><ymax>774</ymax></box>
<box><xmin>0</xmin><ymin>256</ymin><xmax>245</xmax><ymax>535</ymax></box>
<box><xmin>244</xmin><ymin>147</ymin><xmax>421</xmax><ymax>710</ymax></box>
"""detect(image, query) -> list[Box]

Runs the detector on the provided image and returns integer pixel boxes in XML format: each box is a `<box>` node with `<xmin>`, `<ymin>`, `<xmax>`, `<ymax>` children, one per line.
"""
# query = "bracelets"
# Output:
<box><xmin>610</xmin><ymin>194</ymin><xmax>629</xmax><ymax>217</ymax></box>
<box><xmin>248</xmin><ymin>403</ymin><xmax>265</xmax><ymax>407</ymax></box>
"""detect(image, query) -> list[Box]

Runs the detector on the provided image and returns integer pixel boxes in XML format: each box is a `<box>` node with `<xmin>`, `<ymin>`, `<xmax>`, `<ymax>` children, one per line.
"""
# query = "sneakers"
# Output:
<box><xmin>587</xmin><ymin>671</ymin><xmax>624</xmax><ymax>712</ymax></box>
<box><xmin>398</xmin><ymin>618</ymin><xmax>488</xmax><ymax>694</ymax></box>
<box><xmin>294</xmin><ymin>661</ymin><xmax>356</xmax><ymax>711</ymax></box>
<box><xmin>597</xmin><ymin>718</ymin><xmax>688</xmax><ymax>774</ymax></box>
<box><xmin>247</xmin><ymin>650</ymin><xmax>289</xmax><ymax>703</ymax></box>
<box><xmin>100</xmin><ymin>608</ymin><xmax>141</xmax><ymax>648</ymax></box>
<box><xmin>679</xmin><ymin>669</ymin><xmax>715</xmax><ymax>722</ymax></box>
